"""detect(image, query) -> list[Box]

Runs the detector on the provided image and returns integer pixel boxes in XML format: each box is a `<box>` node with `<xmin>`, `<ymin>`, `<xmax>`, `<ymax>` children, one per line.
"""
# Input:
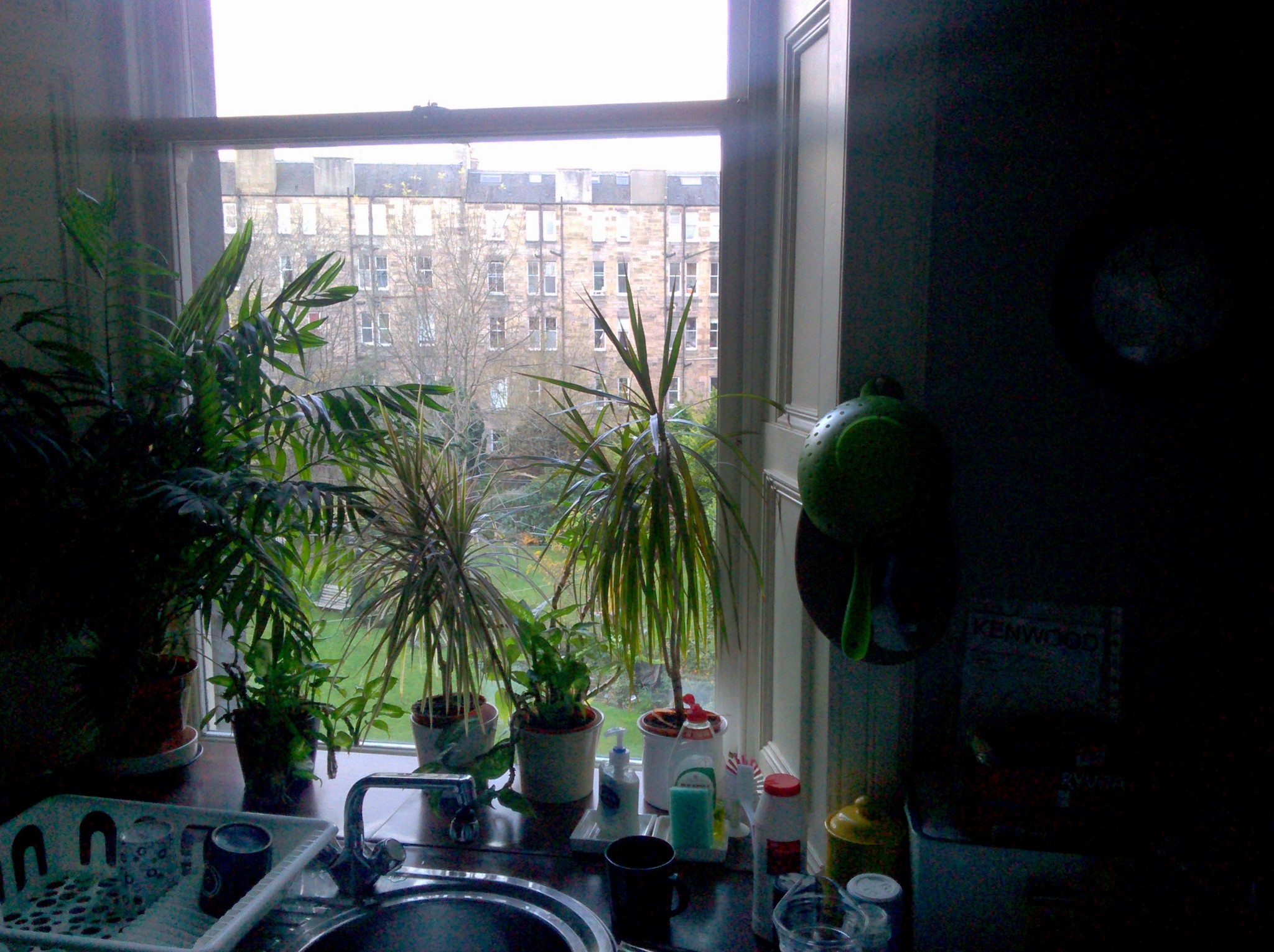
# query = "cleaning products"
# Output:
<box><xmin>668</xmin><ymin>694</ymin><xmax>729</xmax><ymax>843</ymax></box>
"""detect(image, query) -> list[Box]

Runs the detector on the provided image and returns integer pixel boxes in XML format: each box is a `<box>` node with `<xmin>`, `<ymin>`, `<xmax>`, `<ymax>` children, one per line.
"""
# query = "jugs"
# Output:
<box><xmin>772</xmin><ymin>875</ymin><xmax>870</xmax><ymax>952</ymax></box>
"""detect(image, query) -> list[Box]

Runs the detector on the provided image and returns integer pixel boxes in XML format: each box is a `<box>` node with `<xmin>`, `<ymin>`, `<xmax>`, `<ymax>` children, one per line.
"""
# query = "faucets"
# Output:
<box><xmin>316</xmin><ymin>771</ymin><xmax>485</xmax><ymax>893</ymax></box>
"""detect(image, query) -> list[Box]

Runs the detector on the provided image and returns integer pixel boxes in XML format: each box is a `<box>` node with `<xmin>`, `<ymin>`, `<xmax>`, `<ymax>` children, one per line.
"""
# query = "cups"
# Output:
<box><xmin>604</xmin><ymin>836</ymin><xmax>690</xmax><ymax>944</ymax></box>
<box><xmin>199</xmin><ymin>822</ymin><xmax>273</xmax><ymax>919</ymax></box>
<box><xmin>118</xmin><ymin>820</ymin><xmax>179</xmax><ymax>922</ymax></box>
<box><xmin>842</xmin><ymin>873</ymin><xmax>903</xmax><ymax>952</ymax></box>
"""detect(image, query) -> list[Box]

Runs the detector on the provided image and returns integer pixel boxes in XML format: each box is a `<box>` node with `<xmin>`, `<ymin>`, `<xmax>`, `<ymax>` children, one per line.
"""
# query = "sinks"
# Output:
<box><xmin>271</xmin><ymin>869</ymin><xmax>622</xmax><ymax>951</ymax></box>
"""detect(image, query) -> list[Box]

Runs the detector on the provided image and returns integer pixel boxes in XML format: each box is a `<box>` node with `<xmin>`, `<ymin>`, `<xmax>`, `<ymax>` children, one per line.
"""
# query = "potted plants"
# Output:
<box><xmin>483</xmin><ymin>260</ymin><xmax>795</xmax><ymax>814</ymax></box>
<box><xmin>310</xmin><ymin>363</ymin><xmax>604</xmax><ymax>819</ymax></box>
<box><xmin>0</xmin><ymin>175</ymin><xmax>450</xmax><ymax>758</ymax></box>
<box><xmin>483</xmin><ymin>589</ymin><xmax>629</xmax><ymax>809</ymax></box>
<box><xmin>174</xmin><ymin>604</ymin><xmax>407</xmax><ymax>809</ymax></box>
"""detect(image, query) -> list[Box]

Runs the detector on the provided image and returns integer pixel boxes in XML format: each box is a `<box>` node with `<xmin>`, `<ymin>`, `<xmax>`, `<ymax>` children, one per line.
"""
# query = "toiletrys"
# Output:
<box><xmin>595</xmin><ymin>728</ymin><xmax>642</xmax><ymax>836</ymax></box>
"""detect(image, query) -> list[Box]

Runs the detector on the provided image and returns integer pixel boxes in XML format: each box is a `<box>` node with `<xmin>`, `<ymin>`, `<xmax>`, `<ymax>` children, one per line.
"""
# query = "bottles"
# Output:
<box><xmin>667</xmin><ymin>694</ymin><xmax>726</xmax><ymax>850</ymax></box>
<box><xmin>752</xmin><ymin>773</ymin><xmax>802</xmax><ymax>941</ymax></box>
